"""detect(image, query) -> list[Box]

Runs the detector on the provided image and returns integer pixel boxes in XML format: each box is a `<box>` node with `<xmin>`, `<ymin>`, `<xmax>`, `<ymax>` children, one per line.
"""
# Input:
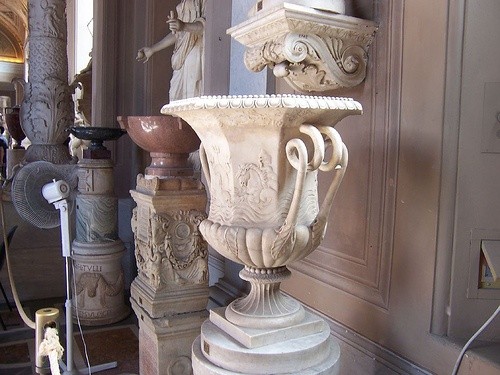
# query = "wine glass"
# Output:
<box><xmin>5</xmin><ymin>107</ymin><xmax>27</xmax><ymax>148</ymax></box>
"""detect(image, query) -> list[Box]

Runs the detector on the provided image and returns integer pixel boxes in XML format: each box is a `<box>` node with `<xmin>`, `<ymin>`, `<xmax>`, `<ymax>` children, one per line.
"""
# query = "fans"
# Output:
<box><xmin>11</xmin><ymin>160</ymin><xmax>118</xmax><ymax>375</ymax></box>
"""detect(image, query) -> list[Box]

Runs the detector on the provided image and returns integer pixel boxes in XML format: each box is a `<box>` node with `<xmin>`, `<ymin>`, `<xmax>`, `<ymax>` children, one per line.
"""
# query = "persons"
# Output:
<box><xmin>0</xmin><ymin>126</ymin><xmax>9</xmax><ymax>166</ymax></box>
<box><xmin>136</xmin><ymin>0</ymin><xmax>205</xmax><ymax>104</ymax></box>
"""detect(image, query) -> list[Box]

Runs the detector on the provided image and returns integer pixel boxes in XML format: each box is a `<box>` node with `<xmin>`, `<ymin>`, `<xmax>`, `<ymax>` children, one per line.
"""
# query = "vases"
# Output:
<box><xmin>117</xmin><ymin>114</ymin><xmax>201</xmax><ymax>175</ymax></box>
<box><xmin>66</xmin><ymin>125</ymin><xmax>127</xmax><ymax>160</ymax></box>
<box><xmin>5</xmin><ymin>106</ymin><xmax>28</xmax><ymax>149</ymax></box>
<box><xmin>159</xmin><ymin>93</ymin><xmax>366</xmax><ymax>328</ymax></box>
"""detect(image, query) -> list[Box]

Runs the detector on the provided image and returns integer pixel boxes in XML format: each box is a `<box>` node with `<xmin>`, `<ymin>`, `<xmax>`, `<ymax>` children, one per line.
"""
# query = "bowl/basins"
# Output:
<box><xmin>117</xmin><ymin>115</ymin><xmax>201</xmax><ymax>167</ymax></box>
<box><xmin>64</xmin><ymin>127</ymin><xmax>126</xmax><ymax>151</ymax></box>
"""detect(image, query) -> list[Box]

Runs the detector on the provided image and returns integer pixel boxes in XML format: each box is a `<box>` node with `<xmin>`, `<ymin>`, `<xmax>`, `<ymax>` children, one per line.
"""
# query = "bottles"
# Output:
<box><xmin>35</xmin><ymin>308</ymin><xmax>60</xmax><ymax>374</ymax></box>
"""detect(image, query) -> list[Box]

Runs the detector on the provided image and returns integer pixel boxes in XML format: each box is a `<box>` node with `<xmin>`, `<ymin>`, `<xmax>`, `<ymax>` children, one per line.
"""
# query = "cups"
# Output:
<box><xmin>161</xmin><ymin>93</ymin><xmax>367</xmax><ymax>330</ymax></box>
